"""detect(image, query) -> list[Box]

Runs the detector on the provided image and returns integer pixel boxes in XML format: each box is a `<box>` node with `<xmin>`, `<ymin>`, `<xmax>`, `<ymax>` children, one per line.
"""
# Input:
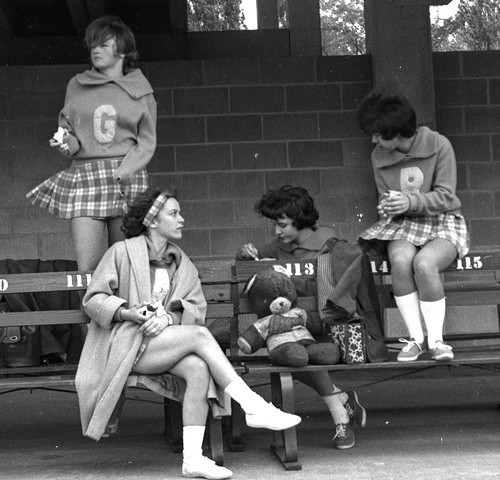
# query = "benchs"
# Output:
<box><xmin>0</xmin><ymin>252</ymin><xmax>500</xmax><ymax>470</ymax></box>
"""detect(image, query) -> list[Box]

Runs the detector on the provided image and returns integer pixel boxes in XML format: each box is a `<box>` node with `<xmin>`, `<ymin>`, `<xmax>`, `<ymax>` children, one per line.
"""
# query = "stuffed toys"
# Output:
<box><xmin>237</xmin><ymin>269</ymin><xmax>340</xmax><ymax>367</ymax></box>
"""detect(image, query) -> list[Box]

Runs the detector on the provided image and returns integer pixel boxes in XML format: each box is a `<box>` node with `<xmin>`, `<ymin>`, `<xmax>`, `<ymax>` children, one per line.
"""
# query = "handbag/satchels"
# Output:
<box><xmin>0</xmin><ymin>326</ymin><xmax>40</xmax><ymax>368</ymax></box>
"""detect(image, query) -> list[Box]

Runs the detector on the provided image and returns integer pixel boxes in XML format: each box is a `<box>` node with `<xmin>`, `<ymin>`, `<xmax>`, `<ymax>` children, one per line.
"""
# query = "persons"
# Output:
<box><xmin>235</xmin><ymin>185</ymin><xmax>388</xmax><ymax>449</ymax></box>
<box><xmin>75</xmin><ymin>187</ymin><xmax>302</xmax><ymax>479</ymax></box>
<box><xmin>357</xmin><ymin>94</ymin><xmax>470</xmax><ymax>362</ymax></box>
<box><xmin>26</xmin><ymin>15</ymin><xmax>157</xmax><ymax>338</ymax></box>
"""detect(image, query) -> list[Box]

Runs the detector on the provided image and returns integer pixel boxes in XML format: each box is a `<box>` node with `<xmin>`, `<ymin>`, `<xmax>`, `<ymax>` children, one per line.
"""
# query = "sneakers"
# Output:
<box><xmin>396</xmin><ymin>338</ymin><xmax>428</xmax><ymax>362</ymax></box>
<box><xmin>428</xmin><ymin>342</ymin><xmax>454</xmax><ymax>361</ymax></box>
<box><xmin>246</xmin><ymin>402</ymin><xmax>301</xmax><ymax>430</ymax></box>
<box><xmin>181</xmin><ymin>457</ymin><xmax>233</xmax><ymax>479</ymax></box>
<box><xmin>332</xmin><ymin>416</ymin><xmax>355</xmax><ymax>450</ymax></box>
<box><xmin>343</xmin><ymin>390</ymin><xmax>366</xmax><ymax>429</ymax></box>
